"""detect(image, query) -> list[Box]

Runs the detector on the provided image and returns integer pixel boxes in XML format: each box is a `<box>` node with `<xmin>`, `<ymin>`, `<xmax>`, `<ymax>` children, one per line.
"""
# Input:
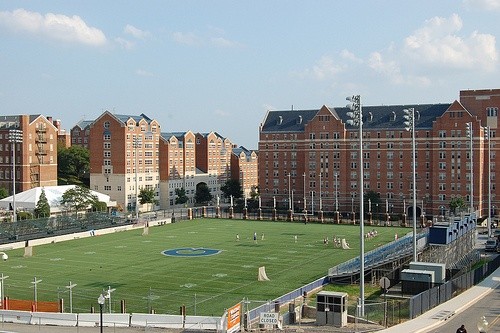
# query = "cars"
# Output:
<box><xmin>485</xmin><ymin>227</ymin><xmax>500</xmax><ymax>253</ymax></box>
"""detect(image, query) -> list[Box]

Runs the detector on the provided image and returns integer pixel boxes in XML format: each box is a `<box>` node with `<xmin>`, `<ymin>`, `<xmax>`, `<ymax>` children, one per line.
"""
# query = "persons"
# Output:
<box><xmin>456</xmin><ymin>325</ymin><xmax>467</xmax><ymax>333</ymax></box>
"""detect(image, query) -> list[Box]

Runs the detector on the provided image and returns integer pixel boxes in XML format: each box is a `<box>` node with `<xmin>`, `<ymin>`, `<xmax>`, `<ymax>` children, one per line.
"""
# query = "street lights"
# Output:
<box><xmin>485</xmin><ymin>125</ymin><xmax>492</xmax><ymax>238</ymax></box>
<box><xmin>98</xmin><ymin>293</ymin><xmax>105</xmax><ymax>333</ymax></box>
<box><xmin>1</xmin><ymin>273</ymin><xmax>9</xmax><ymax>309</ymax></box>
<box><xmin>465</xmin><ymin>122</ymin><xmax>474</xmax><ymax>221</ymax></box>
<box><xmin>302</xmin><ymin>172</ymin><xmax>305</xmax><ymax>211</ymax></box>
<box><xmin>105</xmin><ymin>285</ymin><xmax>116</xmax><ymax>314</ymax></box>
<box><xmin>403</xmin><ymin>107</ymin><xmax>418</xmax><ymax>264</ymax></box>
<box><xmin>66</xmin><ymin>281</ymin><xmax>78</xmax><ymax>313</ymax></box>
<box><xmin>287</xmin><ymin>172</ymin><xmax>292</xmax><ymax>210</ymax></box>
<box><xmin>30</xmin><ymin>277</ymin><xmax>43</xmax><ymax>312</ymax></box>
<box><xmin>333</xmin><ymin>172</ymin><xmax>337</xmax><ymax>212</ymax></box>
<box><xmin>318</xmin><ymin>172</ymin><xmax>322</xmax><ymax>212</ymax></box>
<box><xmin>8</xmin><ymin>129</ymin><xmax>23</xmax><ymax>223</ymax></box>
<box><xmin>344</xmin><ymin>95</ymin><xmax>366</xmax><ymax>320</ymax></box>
